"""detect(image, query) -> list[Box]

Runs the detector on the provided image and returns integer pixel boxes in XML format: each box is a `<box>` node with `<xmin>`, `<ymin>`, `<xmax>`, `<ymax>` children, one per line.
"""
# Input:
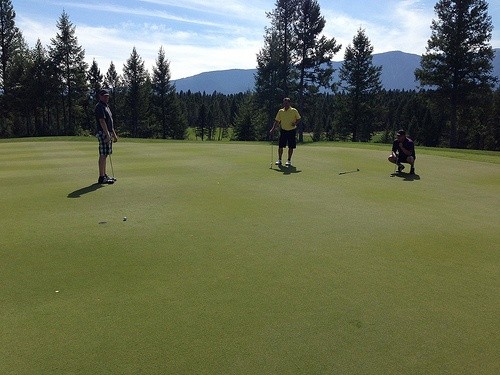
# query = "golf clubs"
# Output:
<box><xmin>339</xmin><ymin>168</ymin><xmax>360</xmax><ymax>175</ymax></box>
<box><xmin>390</xmin><ymin>157</ymin><xmax>398</xmax><ymax>177</ymax></box>
<box><xmin>109</xmin><ymin>154</ymin><xmax>115</xmax><ymax>177</ymax></box>
<box><xmin>270</xmin><ymin>134</ymin><xmax>273</xmax><ymax>168</ymax></box>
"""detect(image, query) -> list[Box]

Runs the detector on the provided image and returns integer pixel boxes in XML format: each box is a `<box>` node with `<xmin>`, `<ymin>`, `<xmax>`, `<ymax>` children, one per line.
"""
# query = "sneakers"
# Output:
<box><xmin>103</xmin><ymin>176</ymin><xmax>117</xmax><ymax>181</ymax></box>
<box><xmin>98</xmin><ymin>178</ymin><xmax>114</xmax><ymax>184</ymax></box>
<box><xmin>285</xmin><ymin>161</ymin><xmax>291</xmax><ymax>165</ymax></box>
<box><xmin>396</xmin><ymin>164</ymin><xmax>405</xmax><ymax>172</ymax></box>
<box><xmin>275</xmin><ymin>160</ymin><xmax>282</xmax><ymax>165</ymax></box>
<box><xmin>409</xmin><ymin>166</ymin><xmax>415</xmax><ymax>175</ymax></box>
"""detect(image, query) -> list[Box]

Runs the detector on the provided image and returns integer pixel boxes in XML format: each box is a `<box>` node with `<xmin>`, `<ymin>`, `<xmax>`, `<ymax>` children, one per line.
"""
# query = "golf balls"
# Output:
<box><xmin>123</xmin><ymin>217</ymin><xmax>127</xmax><ymax>220</ymax></box>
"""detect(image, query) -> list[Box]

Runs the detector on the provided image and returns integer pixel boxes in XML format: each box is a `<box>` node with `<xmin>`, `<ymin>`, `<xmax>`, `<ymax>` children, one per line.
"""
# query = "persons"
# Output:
<box><xmin>93</xmin><ymin>89</ymin><xmax>117</xmax><ymax>184</ymax></box>
<box><xmin>269</xmin><ymin>98</ymin><xmax>301</xmax><ymax>166</ymax></box>
<box><xmin>388</xmin><ymin>130</ymin><xmax>416</xmax><ymax>175</ymax></box>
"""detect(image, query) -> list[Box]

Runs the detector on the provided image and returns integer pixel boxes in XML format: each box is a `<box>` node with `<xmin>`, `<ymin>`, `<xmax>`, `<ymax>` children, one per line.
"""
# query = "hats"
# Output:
<box><xmin>283</xmin><ymin>98</ymin><xmax>291</xmax><ymax>104</ymax></box>
<box><xmin>99</xmin><ymin>89</ymin><xmax>110</xmax><ymax>97</ymax></box>
<box><xmin>397</xmin><ymin>129</ymin><xmax>406</xmax><ymax>136</ymax></box>
